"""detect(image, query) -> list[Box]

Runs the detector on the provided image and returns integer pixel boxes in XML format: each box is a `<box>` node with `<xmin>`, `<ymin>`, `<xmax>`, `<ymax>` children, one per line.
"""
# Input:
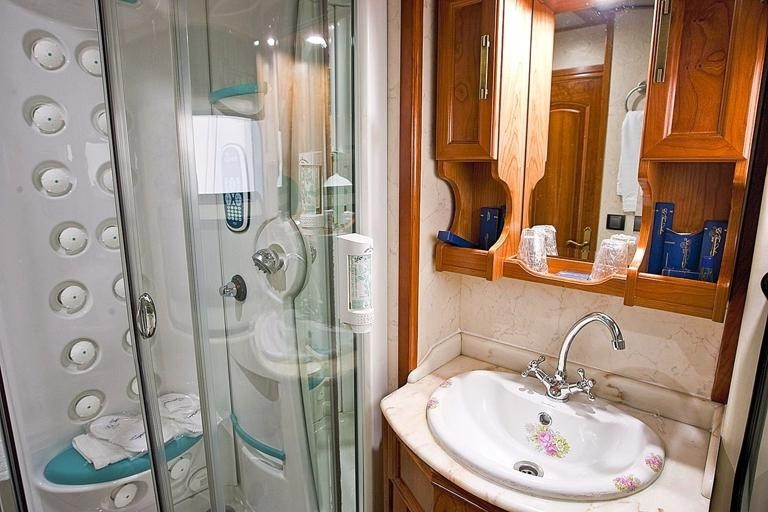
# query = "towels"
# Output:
<box><xmin>69</xmin><ymin>394</ymin><xmax>223</xmax><ymax>471</ymax></box>
<box><xmin>617</xmin><ymin>111</ymin><xmax>644</xmax><ymax>217</ymax></box>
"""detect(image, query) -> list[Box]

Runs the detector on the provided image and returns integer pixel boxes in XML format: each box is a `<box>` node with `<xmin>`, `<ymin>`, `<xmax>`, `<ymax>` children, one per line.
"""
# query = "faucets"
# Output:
<box><xmin>522</xmin><ymin>311</ymin><xmax>626</xmax><ymax>402</ymax></box>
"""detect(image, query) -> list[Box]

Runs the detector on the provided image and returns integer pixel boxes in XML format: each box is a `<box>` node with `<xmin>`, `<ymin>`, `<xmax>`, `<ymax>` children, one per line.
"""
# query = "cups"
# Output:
<box><xmin>610</xmin><ymin>234</ymin><xmax>637</xmax><ymax>266</ymax></box>
<box><xmin>589</xmin><ymin>239</ymin><xmax>629</xmax><ymax>282</ymax></box>
<box><xmin>534</xmin><ymin>223</ymin><xmax>559</xmax><ymax>257</ymax></box>
<box><xmin>515</xmin><ymin>230</ymin><xmax>549</xmax><ymax>275</ymax></box>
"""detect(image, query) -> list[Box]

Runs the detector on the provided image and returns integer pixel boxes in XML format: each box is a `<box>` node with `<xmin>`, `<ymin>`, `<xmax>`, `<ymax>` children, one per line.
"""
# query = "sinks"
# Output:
<box><xmin>425</xmin><ymin>368</ymin><xmax>666</xmax><ymax>512</ymax></box>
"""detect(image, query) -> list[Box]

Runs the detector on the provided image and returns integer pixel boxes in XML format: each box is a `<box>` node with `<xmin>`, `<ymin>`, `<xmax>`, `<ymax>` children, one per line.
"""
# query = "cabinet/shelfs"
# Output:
<box><xmin>430</xmin><ymin>1</ymin><xmax>525</xmax><ymax>280</ymax></box>
<box><xmin>621</xmin><ymin>1</ymin><xmax>767</xmax><ymax>322</ymax></box>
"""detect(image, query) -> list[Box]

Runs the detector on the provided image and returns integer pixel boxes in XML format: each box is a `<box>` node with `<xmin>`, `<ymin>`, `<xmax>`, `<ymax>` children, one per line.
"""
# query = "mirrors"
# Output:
<box><xmin>517</xmin><ymin>0</ymin><xmax>643</xmax><ymax>270</ymax></box>
